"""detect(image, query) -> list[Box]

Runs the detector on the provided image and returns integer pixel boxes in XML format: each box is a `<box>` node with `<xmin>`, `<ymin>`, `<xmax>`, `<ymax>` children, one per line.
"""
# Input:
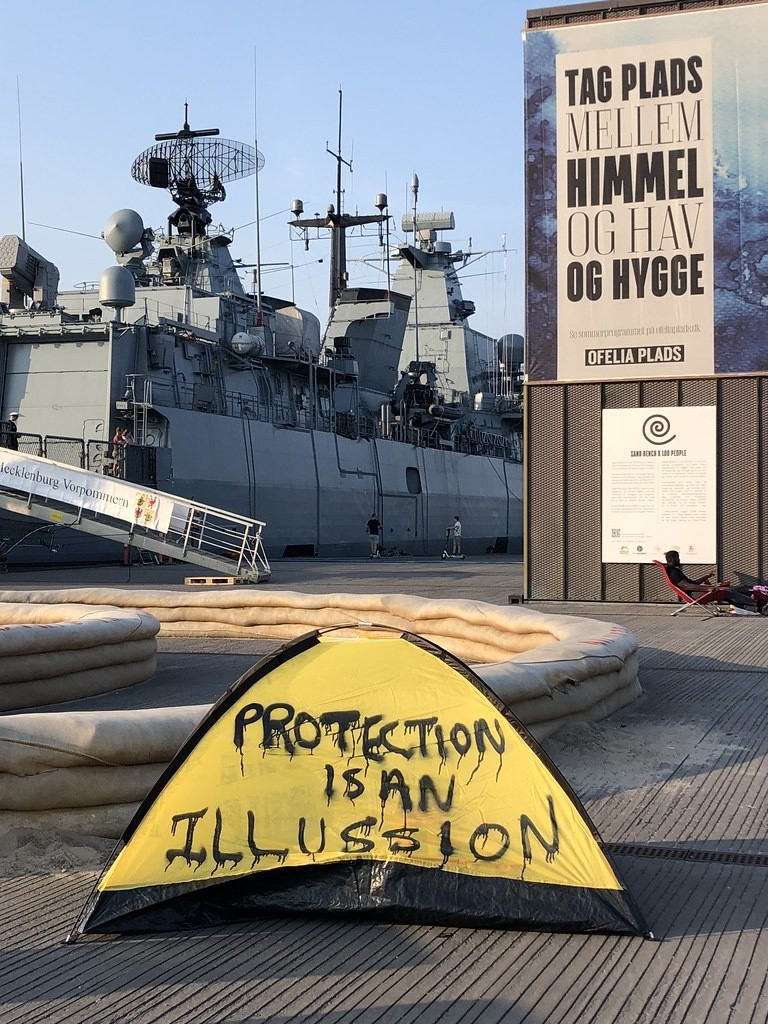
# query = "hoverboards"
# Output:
<box><xmin>441</xmin><ymin>529</ymin><xmax>466</xmax><ymax>561</ymax></box>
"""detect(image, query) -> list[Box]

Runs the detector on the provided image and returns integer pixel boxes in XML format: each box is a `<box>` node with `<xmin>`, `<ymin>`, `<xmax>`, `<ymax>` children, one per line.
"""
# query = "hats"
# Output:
<box><xmin>10</xmin><ymin>412</ymin><xmax>19</xmax><ymax>415</ymax></box>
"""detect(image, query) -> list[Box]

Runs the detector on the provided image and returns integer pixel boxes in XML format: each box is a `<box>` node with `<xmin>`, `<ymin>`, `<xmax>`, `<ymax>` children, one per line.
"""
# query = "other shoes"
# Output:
<box><xmin>761</xmin><ymin>603</ymin><xmax>768</xmax><ymax>616</ymax></box>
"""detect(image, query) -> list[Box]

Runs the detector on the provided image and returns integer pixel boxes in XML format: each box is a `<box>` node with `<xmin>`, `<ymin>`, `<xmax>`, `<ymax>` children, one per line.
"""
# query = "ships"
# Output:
<box><xmin>0</xmin><ymin>40</ymin><xmax>527</xmax><ymax>567</ymax></box>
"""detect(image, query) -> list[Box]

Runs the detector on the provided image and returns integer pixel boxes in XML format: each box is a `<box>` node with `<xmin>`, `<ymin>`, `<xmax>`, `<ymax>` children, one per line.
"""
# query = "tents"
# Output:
<box><xmin>67</xmin><ymin>621</ymin><xmax>655</xmax><ymax>943</ymax></box>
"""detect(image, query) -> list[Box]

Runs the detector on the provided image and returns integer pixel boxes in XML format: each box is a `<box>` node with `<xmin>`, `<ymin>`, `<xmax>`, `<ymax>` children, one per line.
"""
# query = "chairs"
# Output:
<box><xmin>653</xmin><ymin>560</ymin><xmax>730</xmax><ymax>616</ymax></box>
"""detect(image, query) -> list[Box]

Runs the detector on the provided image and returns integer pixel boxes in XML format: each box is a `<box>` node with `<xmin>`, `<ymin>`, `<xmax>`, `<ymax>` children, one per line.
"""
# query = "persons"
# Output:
<box><xmin>366</xmin><ymin>514</ymin><xmax>381</xmax><ymax>556</ymax></box>
<box><xmin>447</xmin><ymin>516</ymin><xmax>461</xmax><ymax>556</ymax></box>
<box><xmin>666</xmin><ymin>550</ymin><xmax>768</xmax><ymax>615</ymax></box>
<box><xmin>188</xmin><ymin>509</ymin><xmax>201</xmax><ymax>548</ymax></box>
<box><xmin>112</xmin><ymin>427</ymin><xmax>124</xmax><ymax>476</ymax></box>
<box><xmin>5</xmin><ymin>411</ymin><xmax>21</xmax><ymax>451</ymax></box>
<box><xmin>121</xmin><ymin>428</ymin><xmax>133</xmax><ymax>445</ymax></box>
